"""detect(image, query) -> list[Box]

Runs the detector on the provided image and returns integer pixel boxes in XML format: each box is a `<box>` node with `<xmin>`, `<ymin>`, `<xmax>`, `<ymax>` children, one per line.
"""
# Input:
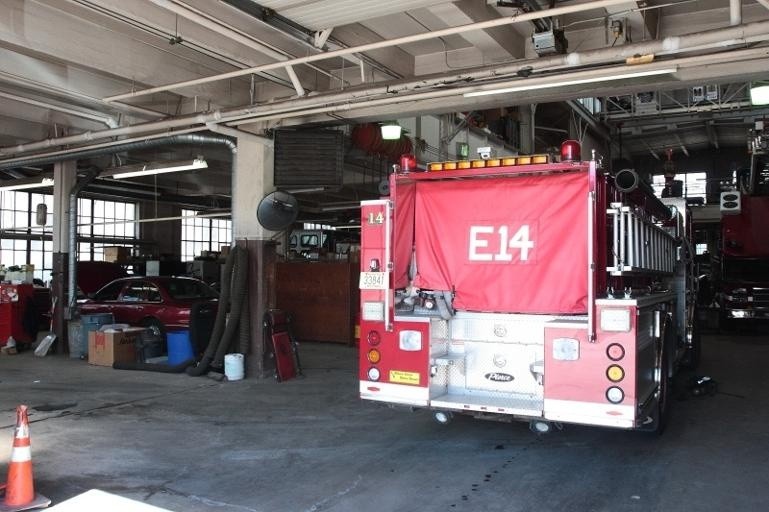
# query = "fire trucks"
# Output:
<box><xmin>716</xmin><ymin>169</ymin><xmax>769</xmax><ymax>325</ymax></box>
<box><xmin>358</xmin><ymin>139</ymin><xmax>710</xmax><ymax>436</ymax></box>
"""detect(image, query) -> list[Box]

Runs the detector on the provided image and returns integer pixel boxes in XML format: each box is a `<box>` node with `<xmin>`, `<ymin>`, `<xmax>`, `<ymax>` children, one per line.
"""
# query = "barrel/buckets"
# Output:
<box><xmin>224</xmin><ymin>354</ymin><xmax>245</xmax><ymax>381</ymax></box>
<box><xmin>166</xmin><ymin>329</ymin><xmax>192</xmax><ymax>367</ymax></box>
<box><xmin>67</xmin><ymin>321</ymin><xmax>100</xmax><ymax>358</ymax></box>
<box><xmin>80</xmin><ymin>312</ymin><xmax>113</xmax><ymax>324</ymax></box>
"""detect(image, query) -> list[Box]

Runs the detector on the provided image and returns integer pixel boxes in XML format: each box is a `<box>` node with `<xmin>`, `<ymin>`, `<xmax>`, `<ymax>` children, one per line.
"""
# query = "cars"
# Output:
<box><xmin>49</xmin><ymin>275</ymin><xmax>231</xmax><ymax>355</ymax></box>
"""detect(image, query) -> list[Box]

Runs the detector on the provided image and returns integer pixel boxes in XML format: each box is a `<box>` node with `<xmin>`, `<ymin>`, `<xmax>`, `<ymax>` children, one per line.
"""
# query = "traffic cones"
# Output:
<box><xmin>0</xmin><ymin>404</ymin><xmax>52</xmax><ymax>512</ymax></box>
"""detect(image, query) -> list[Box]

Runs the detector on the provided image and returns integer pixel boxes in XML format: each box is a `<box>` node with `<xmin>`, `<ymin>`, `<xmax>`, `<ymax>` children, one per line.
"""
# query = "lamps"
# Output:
<box><xmin>1</xmin><ymin>171</ymin><xmax>55</xmax><ymax>192</ymax></box>
<box><xmin>97</xmin><ymin>147</ymin><xmax>209</xmax><ymax>181</ymax></box>
